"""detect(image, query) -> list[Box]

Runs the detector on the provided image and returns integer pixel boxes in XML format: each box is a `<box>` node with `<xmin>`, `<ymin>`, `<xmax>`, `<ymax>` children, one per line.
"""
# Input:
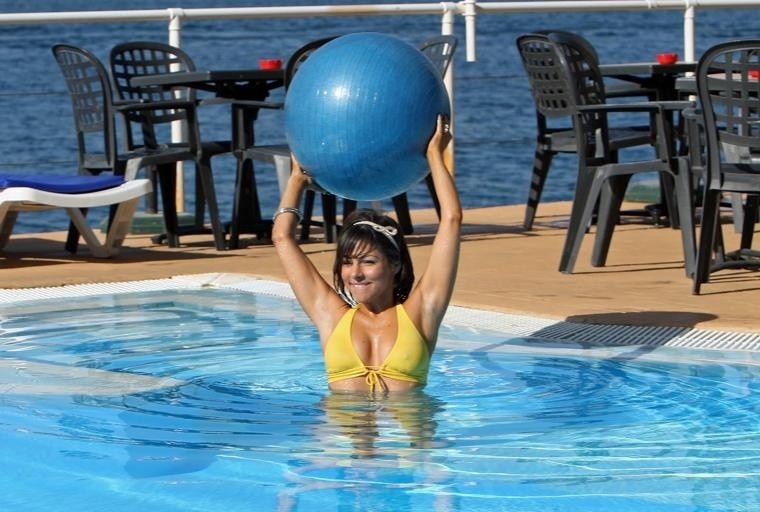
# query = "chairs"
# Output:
<box><xmin>691</xmin><ymin>39</ymin><xmax>759</xmax><ymax>296</ymax></box>
<box><xmin>520</xmin><ymin>36</ymin><xmax>661</xmax><ymax>233</ymax></box>
<box><xmin>413</xmin><ymin>36</ymin><xmax>459</xmax><ymax>223</ymax></box>
<box><xmin>109</xmin><ymin>38</ymin><xmax>239</xmax><ymax>235</ymax></box>
<box><xmin>551</xmin><ymin>39</ymin><xmax>698</xmax><ymax>277</ymax></box>
<box><xmin>1</xmin><ymin>171</ymin><xmax>154</xmax><ymax>260</ymax></box>
<box><xmin>225</xmin><ymin>36</ymin><xmax>417</xmax><ymax>245</ymax></box>
<box><xmin>50</xmin><ymin>42</ymin><xmax>226</xmax><ymax>250</ymax></box>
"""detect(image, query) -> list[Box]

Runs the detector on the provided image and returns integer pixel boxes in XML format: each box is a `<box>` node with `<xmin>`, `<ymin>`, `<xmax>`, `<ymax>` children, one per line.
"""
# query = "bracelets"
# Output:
<box><xmin>272</xmin><ymin>207</ymin><xmax>302</xmax><ymax>224</ymax></box>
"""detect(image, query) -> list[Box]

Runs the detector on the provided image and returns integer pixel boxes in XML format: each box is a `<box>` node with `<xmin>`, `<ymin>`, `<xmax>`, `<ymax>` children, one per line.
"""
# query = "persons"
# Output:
<box><xmin>273</xmin><ymin>110</ymin><xmax>461</xmax><ymax>397</ymax></box>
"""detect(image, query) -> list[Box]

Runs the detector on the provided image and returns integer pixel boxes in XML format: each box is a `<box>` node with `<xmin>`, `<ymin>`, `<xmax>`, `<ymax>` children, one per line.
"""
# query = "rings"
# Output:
<box><xmin>444</xmin><ymin>124</ymin><xmax>450</xmax><ymax>133</ymax></box>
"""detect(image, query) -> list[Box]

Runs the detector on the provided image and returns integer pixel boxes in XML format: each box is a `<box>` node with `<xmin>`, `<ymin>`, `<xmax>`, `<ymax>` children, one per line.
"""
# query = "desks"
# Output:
<box><xmin>582</xmin><ymin>58</ymin><xmax>756</xmax><ymax>228</ymax></box>
<box><xmin>129</xmin><ymin>69</ymin><xmax>297</xmax><ymax>249</ymax></box>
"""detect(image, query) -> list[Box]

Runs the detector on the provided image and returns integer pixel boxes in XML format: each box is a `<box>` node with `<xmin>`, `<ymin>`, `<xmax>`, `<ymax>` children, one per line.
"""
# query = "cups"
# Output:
<box><xmin>257</xmin><ymin>58</ymin><xmax>284</xmax><ymax>69</ymax></box>
<box><xmin>654</xmin><ymin>52</ymin><xmax>680</xmax><ymax>64</ymax></box>
